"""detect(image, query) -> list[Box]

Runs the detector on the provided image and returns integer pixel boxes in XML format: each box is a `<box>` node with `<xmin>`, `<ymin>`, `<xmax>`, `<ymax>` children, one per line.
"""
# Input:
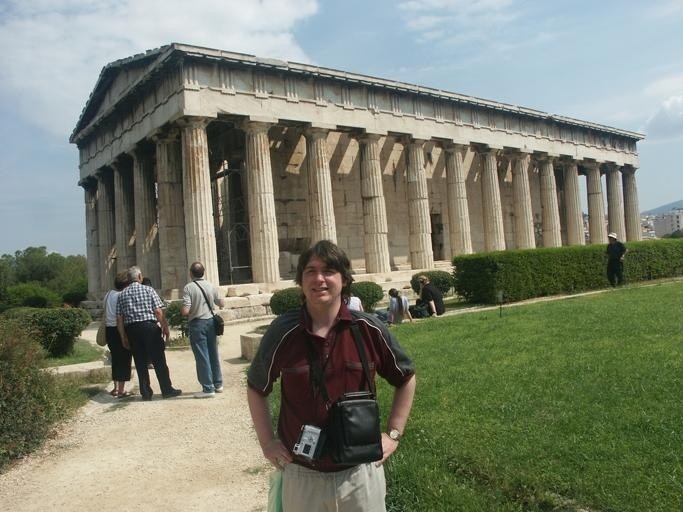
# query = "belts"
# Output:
<box><xmin>128</xmin><ymin>321</ymin><xmax>155</xmax><ymax>328</ymax></box>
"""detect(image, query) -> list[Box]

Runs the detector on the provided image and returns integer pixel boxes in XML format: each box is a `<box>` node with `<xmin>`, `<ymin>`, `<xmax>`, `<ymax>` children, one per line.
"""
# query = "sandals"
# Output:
<box><xmin>110</xmin><ymin>389</ymin><xmax>119</xmax><ymax>396</ymax></box>
<box><xmin>118</xmin><ymin>391</ymin><xmax>134</xmax><ymax>398</ymax></box>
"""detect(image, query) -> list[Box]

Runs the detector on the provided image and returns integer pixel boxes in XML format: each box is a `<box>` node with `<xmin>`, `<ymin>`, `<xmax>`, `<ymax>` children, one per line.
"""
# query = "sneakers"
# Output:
<box><xmin>215</xmin><ymin>385</ymin><xmax>223</xmax><ymax>393</ymax></box>
<box><xmin>163</xmin><ymin>389</ymin><xmax>181</xmax><ymax>398</ymax></box>
<box><xmin>193</xmin><ymin>391</ymin><xmax>216</xmax><ymax>398</ymax></box>
<box><xmin>142</xmin><ymin>395</ymin><xmax>151</xmax><ymax>401</ymax></box>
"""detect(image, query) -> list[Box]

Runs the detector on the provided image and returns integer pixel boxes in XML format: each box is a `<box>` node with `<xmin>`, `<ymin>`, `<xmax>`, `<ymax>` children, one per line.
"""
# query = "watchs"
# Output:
<box><xmin>386</xmin><ymin>427</ymin><xmax>401</xmax><ymax>441</ymax></box>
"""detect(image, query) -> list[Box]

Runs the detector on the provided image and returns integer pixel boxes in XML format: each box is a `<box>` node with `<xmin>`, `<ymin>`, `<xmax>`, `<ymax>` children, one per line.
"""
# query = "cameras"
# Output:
<box><xmin>292</xmin><ymin>424</ymin><xmax>326</xmax><ymax>461</ymax></box>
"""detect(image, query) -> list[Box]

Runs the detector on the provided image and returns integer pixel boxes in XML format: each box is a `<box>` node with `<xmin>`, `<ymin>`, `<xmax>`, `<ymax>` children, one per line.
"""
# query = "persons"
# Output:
<box><xmin>247</xmin><ymin>240</ymin><xmax>416</xmax><ymax>512</ymax></box>
<box><xmin>102</xmin><ymin>262</ymin><xmax>225</xmax><ymax>400</ymax></box>
<box><xmin>344</xmin><ymin>292</ymin><xmax>364</xmax><ymax>312</ymax></box>
<box><xmin>606</xmin><ymin>232</ymin><xmax>628</xmax><ymax>289</ymax></box>
<box><xmin>417</xmin><ymin>275</ymin><xmax>445</xmax><ymax>317</ymax></box>
<box><xmin>387</xmin><ymin>289</ymin><xmax>412</xmax><ymax>322</ymax></box>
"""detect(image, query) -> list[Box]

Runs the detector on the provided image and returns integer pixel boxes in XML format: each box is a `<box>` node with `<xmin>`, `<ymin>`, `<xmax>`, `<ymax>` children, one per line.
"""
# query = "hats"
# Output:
<box><xmin>608</xmin><ymin>233</ymin><xmax>617</xmax><ymax>240</ymax></box>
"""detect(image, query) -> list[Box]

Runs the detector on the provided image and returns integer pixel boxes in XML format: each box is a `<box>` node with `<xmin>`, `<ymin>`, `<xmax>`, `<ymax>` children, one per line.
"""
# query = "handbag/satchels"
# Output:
<box><xmin>213</xmin><ymin>315</ymin><xmax>224</xmax><ymax>335</ymax></box>
<box><xmin>408</xmin><ymin>302</ymin><xmax>430</xmax><ymax>318</ymax></box>
<box><xmin>97</xmin><ymin>325</ymin><xmax>106</xmax><ymax>346</ymax></box>
<box><xmin>324</xmin><ymin>391</ymin><xmax>383</xmax><ymax>464</ymax></box>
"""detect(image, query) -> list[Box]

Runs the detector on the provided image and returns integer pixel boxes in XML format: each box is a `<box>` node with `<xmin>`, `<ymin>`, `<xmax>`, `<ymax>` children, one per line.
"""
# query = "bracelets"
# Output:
<box><xmin>433</xmin><ymin>312</ymin><xmax>437</xmax><ymax>313</ymax></box>
